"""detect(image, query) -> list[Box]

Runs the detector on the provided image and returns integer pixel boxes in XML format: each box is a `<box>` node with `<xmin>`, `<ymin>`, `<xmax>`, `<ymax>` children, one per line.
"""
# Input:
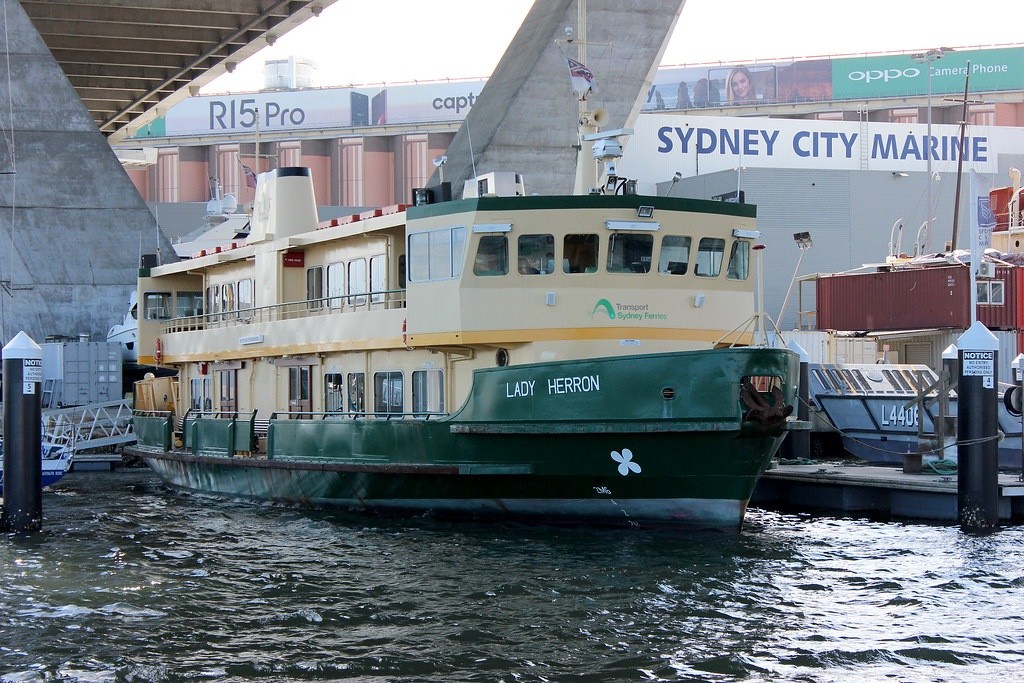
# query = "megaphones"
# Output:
<box><xmin>588</xmin><ymin>108</ymin><xmax>609</xmax><ymax>127</ymax></box>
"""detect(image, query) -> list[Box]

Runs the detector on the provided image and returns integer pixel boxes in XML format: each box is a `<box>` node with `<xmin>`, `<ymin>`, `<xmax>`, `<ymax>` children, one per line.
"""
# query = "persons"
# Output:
<box><xmin>726</xmin><ymin>66</ymin><xmax>757</xmax><ymax>105</ymax></box>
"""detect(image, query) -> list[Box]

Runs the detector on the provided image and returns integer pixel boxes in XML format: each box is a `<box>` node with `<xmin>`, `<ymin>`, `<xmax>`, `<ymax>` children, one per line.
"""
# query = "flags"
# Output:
<box><xmin>566</xmin><ymin>58</ymin><xmax>599</xmax><ymax>94</ymax></box>
<box><xmin>244</xmin><ymin>166</ymin><xmax>257</xmax><ymax>189</ymax></box>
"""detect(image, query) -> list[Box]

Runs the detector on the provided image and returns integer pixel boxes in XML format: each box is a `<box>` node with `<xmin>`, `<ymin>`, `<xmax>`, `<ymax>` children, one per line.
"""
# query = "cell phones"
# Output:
<box><xmin>707</xmin><ymin>67</ymin><xmax>778</xmax><ymax>101</ymax></box>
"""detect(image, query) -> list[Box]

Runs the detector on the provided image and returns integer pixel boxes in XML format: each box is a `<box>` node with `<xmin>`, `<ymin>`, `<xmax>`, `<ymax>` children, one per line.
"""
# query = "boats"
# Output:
<box><xmin>0</xmin><ymin>414</ymin><xmax>76</xmax><ymax>494</ymax></box>
<box><xmin>123</xmin><ymin>1</ymin><xmax>803</xmax><ymax>545</ymax></box>
<box><xmin>806</xmin><ymin>344</ymin><xmax>1024</xmax><ymax>475</ymax></box>
<box><xmin>105</xmin><ymin>288</ymin><xmax>220</xmax><ymax>363</ymax></box>
